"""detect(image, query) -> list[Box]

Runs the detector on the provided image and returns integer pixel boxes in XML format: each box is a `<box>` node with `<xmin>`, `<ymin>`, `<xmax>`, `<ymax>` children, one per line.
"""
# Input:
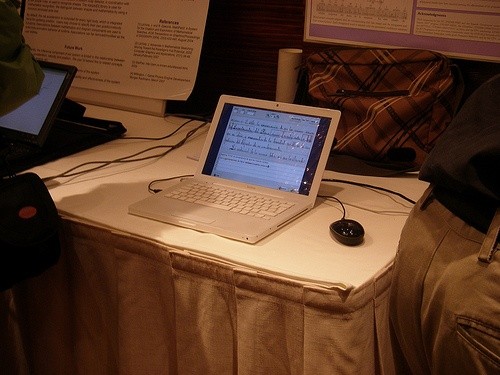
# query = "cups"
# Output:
<box><xmin>276</xmin><ymin>49</ymin><xmax>303</xmax><ymax>103</ymax></box>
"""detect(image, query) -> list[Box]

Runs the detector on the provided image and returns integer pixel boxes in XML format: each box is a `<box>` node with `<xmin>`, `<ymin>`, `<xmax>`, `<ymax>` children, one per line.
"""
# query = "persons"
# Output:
<box><xmin>0</xmin><ymin>0</ymin><xmax>46</xmax><ymax>118</ymax></box>
<box><xmin>389</xmin><ymin>74</ymin><xmax>500</xmax><ymax>375</ymax></box>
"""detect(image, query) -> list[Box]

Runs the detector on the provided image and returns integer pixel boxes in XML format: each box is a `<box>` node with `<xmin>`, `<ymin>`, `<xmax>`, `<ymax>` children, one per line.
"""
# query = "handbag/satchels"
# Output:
<box><xmin>293</xmin><ymin>46</ymin><xmax>469</xmax><ymax>171</ymax></box>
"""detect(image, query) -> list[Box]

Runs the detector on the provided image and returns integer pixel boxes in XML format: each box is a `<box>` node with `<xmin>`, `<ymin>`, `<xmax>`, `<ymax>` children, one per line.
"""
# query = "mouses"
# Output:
<box><xmin>330</xmin><ymin>220</ymin><xmax>366</xmax><ymax>246</ymax></box>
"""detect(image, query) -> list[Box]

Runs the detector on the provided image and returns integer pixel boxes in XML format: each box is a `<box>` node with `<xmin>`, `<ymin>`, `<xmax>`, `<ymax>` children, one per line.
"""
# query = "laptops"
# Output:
<box><xmin>128</xmin><ymin>94</ymin><xmax>341</xmax><ymax>244</ymax></box>
<box><xmin>0</xmin><ymin>57</ymin><xmax>76</xmax><ymax>163</ymax></box>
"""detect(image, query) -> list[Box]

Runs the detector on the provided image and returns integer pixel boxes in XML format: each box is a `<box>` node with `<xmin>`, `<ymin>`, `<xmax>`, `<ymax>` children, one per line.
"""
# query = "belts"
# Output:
<box><xmin>429</xmin><ymin>165</ymin><xmax>500</xmax><ymax>242</ymax></box>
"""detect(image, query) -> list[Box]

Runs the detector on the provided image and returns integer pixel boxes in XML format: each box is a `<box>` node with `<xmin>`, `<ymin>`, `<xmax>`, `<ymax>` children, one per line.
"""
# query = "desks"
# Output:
<box><xmin>14</xmin><ymin>95</ymin><xmax>431</xmax><ymax>374</ymax></box>
